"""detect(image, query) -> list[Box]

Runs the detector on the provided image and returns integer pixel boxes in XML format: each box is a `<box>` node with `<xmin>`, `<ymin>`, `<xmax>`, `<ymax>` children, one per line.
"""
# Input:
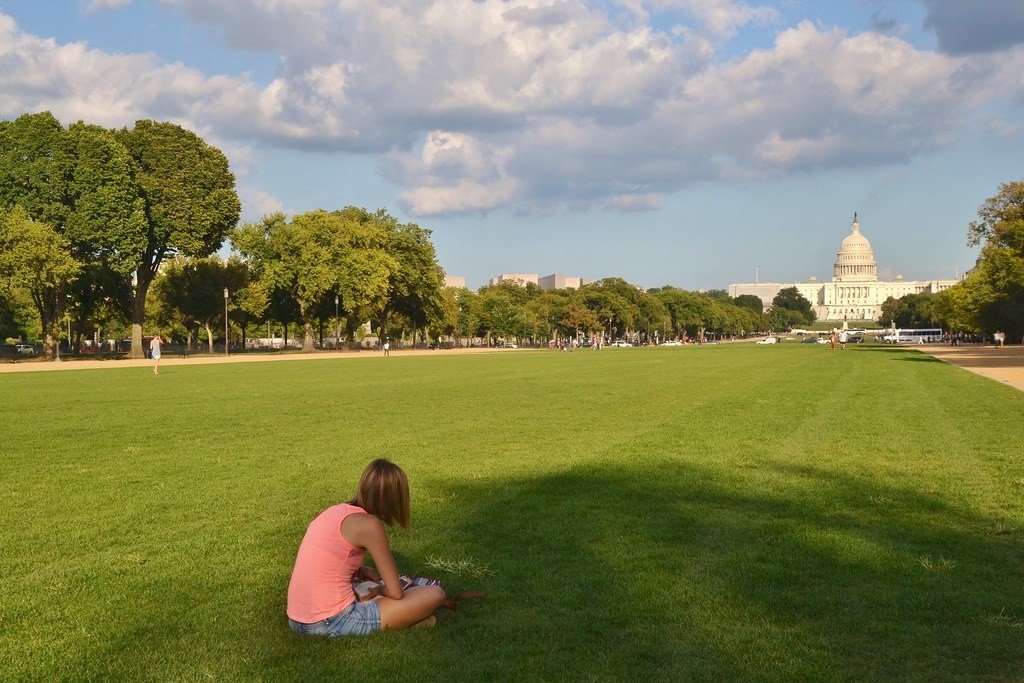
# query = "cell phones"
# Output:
<box><xmin>399</xmin><ymin>576</ymin><xmax>413</xmax><ymax>590</ymax></box>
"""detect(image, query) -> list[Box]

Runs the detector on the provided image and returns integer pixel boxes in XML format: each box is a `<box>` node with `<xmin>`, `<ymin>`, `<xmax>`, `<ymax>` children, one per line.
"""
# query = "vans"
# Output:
<box><xmin>756</xmin><ymin>337</ymin><xmax>776</xmax><ymax>344</ymax></box>
<box><xmin>15</xmin><ymin>344</ymin><xmax>34</xmax><ymax>357</ymax></box>
<box><xmin>847</xmin><ymin>336</ymin><xmax>864</xmax><ymax>343</ymax></box>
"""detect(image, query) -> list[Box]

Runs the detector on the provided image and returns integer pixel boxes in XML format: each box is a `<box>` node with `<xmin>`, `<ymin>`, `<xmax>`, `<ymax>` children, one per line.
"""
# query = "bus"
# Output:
<box><xmin>883</xmin><ymin>329</ymin><xmax>943</xmax><ymax>344</ymax></box>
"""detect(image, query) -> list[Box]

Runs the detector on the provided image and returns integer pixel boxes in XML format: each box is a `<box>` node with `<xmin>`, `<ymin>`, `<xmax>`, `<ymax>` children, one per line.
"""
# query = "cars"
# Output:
<box><xmin>660</xmin><ymin>340</ymin><xmax>682</xmax><ymax>346</ymax></box>
<box><xmin>505</xmin><ymin>343</ymin><xmax>519</xmax><ymax>348</ymax></box>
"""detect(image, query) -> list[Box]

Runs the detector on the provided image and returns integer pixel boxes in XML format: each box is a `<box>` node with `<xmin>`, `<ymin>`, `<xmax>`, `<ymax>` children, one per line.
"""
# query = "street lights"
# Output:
<box><xmin>609</xmin><ymin>318</ymin><xmax>612</xmax><ymax>340</ymax></box>
<box><xmin>335</xmin><ymin>294</ymin><xmax>339</xmax><ymax>351</ymax></box>
<box><xmin>224</xmin><ymin>287</ymin><xmax>229</xmax><ymax>357</ymax></box>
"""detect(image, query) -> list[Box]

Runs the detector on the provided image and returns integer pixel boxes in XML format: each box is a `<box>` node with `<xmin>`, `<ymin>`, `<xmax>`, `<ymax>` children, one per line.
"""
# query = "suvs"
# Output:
<box><xmin>610</xmin><ymin>340</ymin><xmax>632</xmax><ymax>348</ymax></box>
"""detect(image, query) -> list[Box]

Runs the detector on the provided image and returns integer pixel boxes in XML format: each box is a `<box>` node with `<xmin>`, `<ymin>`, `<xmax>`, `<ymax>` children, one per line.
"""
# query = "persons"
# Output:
<box><xmin>829</xmin><ymin>329</ymin><xmax>848</xmax><ymax>350</ymax></box>
<box><xmin>287</xmin><ymin>458</ymin><xmax>446</xmax><ymax>637</ymax></box>
<box><xmin>943</xmin><ymin>331</ymin><xmax>962</xmax><ymax>346</ymax></box>
<box><xmin>548</xmin><ymin>338</ymin><xmax>570</xmax><ymax>352</ymax></box>
<box><xmin>874</xmin><ymin>332</ymin><xmax>887</xmax><ymax>342</ymax></box>
<box><xmin>679</xmin><ymin>337</ymin><xmax>693</xmax><ymax>344</ymax></box>
<box><xmin>573</xmin><ymin>334</ymin><xmax>583</xmax><ymax>349</ymax></box>
<box><xmin>993</xmin><ymin>329</ymin><xmax>1006</xmax><ymax>346</ymax></box>
<box><xmin>150</xmin><ymin>334</ymin><xmax>164</xmax><ymax>374</ymax></box>
<box><xmin>384</xmin><ymin>342</ymin><xmax>389</xmax><ymax>357</ymax></box>
<box><xmin>591</xmin><ymin>335</ymin><xmax>601</xmax><ymax>351</ymax></box>
